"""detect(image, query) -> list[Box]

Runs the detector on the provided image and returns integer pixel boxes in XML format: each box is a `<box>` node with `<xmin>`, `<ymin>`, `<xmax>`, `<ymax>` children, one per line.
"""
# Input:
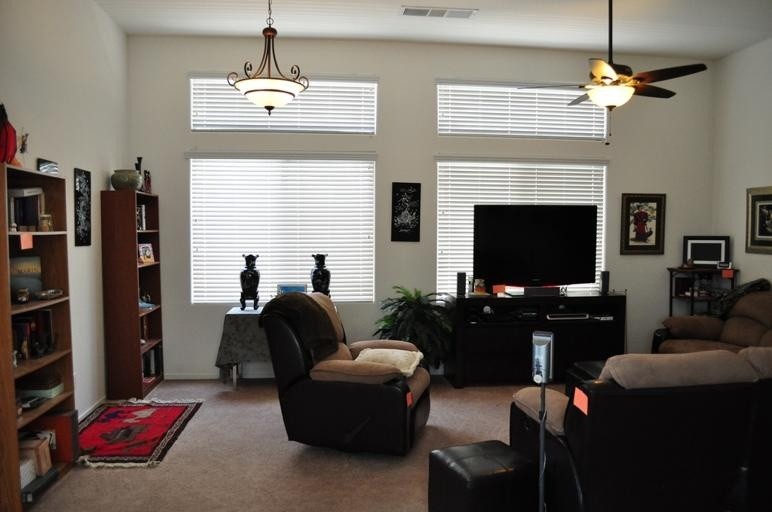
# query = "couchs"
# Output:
<box><xmin>508</xmin><ymin>347</ymin><xmax>772</xmax><ymax>511</ymax></box>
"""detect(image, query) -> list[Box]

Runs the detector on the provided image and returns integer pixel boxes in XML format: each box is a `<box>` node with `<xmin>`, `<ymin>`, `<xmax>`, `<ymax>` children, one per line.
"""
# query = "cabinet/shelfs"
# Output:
<box><xmin>451</xmin><ymin>292</ymin><xmax>626</xmax><ymax>387</ymax></box>
<box><xmin>0</xmin><ymin>162</ymin><xmax>81</xmax><ymax>511</ymax></box>
<box><xmin>100</xmin><ymin>189</ymin><xmax>165</xmax><ymax>402</ymax></box>
<box><xmin>666</xmin><ymin>266</ymin><xmax>740</xmax><ymax>318</ymax></box>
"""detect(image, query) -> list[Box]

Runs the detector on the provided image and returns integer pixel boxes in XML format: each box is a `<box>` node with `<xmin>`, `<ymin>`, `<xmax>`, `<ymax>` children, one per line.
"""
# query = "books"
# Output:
<box><xmin>17</xmin><ymin>377</ymin><xmax>64</xmax><ymax>399</ymax></box>
<box><xmin>142</xmin><ymin>344</ymin><xmax>160</xmax><ymax>380</ymax></box>
<box><xmin>8</xmin><ymin>185</ymin><xmax>46</xmax><ymax>231</ymax></box>
<box><xmin>137</xmin><ymin>204</ymin><xmax>146</xmax><ymax>230</ymax></box>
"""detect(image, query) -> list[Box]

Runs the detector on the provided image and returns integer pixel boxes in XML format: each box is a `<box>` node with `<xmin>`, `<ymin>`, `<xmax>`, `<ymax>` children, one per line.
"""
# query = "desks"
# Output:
<box><xmin>214</xmin><ymin>306</ymin><xmax>276</xmax><ymax>392</ymax></box>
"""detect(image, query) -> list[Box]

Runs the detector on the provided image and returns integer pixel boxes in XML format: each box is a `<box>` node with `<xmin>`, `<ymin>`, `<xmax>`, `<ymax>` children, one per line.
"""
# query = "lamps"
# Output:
<box><xmin>586</xmin><ymin>86</ymin><xmax>636</xmax><ymax>111</ymax></box>
<box><xmin>227</xmin><ymin>0</ymin><xmax>309</xmax><ymax>116</ymax></box>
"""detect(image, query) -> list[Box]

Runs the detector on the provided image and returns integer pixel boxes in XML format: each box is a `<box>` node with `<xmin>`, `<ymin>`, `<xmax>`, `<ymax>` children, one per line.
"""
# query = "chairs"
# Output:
<box><xmin>650</xmin><ymin>277</ymin><xmax>772</xmax><ymax>353</ymax></box>
<box><xmin>259</xmin><ymin>292</ymin><xmax>431</xmax><ymax>457</ymax></box>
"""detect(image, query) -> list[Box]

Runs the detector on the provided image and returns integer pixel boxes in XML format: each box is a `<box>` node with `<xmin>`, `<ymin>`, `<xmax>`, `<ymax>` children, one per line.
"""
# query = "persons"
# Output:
<box><xmin>633</xmin><ymin>205</ymin><xmax>648</xmax><ymax>243</ymax></box>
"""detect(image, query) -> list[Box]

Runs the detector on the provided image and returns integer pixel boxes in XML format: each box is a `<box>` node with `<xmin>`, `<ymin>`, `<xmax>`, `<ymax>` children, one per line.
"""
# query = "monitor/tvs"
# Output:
<box><xmin>276</xmin><ymin>284</ymin><xmax>308</xmax><ymax>295</ymax></box>
<box><xmin>473</xmin><ymin>202</ymin><xmax>596</xmax><ymax>287</ymax></box>
<box><xmin>686</xmin><ymin>239</ymin><xmax>726</xmax><ymax>267</ymax></box>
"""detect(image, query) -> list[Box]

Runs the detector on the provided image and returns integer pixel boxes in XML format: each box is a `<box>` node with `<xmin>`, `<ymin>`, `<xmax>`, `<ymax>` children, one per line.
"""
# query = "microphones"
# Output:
<box><xmin>530</xmin><ymin>331</ymin><xmax>554</xmax><ymax>384</ymax></box>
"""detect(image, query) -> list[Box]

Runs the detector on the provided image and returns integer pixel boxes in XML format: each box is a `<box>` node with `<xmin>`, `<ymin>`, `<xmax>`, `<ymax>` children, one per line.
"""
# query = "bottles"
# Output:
<box><xmin>474</xmin><ymin>279</ymin><xmax>486</xmax><ymax>293</ymax></box>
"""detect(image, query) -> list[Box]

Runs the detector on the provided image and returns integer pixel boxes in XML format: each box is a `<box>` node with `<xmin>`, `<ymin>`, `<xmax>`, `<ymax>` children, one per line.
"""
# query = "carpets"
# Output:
<box><xmin>74</xmin><ymin>400</ymin><xmax>203</xmax><ymax>471</ymax></box>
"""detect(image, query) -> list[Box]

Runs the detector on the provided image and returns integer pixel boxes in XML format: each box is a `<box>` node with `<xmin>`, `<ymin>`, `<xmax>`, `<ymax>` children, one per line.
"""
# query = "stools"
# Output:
<box><xmin>427</xmin><ymin>438</ymin><xmax>535</xmax><ymax>512</ymax></box>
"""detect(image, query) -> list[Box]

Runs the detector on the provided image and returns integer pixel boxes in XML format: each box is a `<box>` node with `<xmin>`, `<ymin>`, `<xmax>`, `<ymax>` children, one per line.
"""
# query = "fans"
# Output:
<box><xmin>517</xmin><ymin>0</ymin><xmax>707</xmax><ymax>106</ymax></box>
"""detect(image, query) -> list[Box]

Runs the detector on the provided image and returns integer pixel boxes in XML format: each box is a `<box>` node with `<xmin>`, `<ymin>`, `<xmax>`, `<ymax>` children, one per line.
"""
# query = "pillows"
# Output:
<box><xmin>354</xmin><ymin>347</ymin><xmax>424</xmax><ymax>378</ymax></box>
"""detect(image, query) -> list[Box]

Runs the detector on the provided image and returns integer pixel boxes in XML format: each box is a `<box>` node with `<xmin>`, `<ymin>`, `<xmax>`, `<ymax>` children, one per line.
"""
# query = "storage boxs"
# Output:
<box><xmin>27</xmin><ymin>409</ymin><xmax>80</xmax><ymax>463</ymax></box>
<box><xmin>19</xmin><ymin>438</ymin><xmax>53</xmax><ymax>477</ymax></box>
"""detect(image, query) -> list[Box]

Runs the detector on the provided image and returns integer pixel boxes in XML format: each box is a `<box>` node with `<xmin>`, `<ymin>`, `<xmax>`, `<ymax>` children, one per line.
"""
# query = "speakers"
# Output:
<box><xmin>524</xmin><ymin>287</ymin><xmax>561</xmax><ymax>297</ymax></box>
<box><xmin>599</xmin><ymin>270</ymin><xmax>610</xmax><ymax>295</ymax></box>
<box><xmin>456</xmin><ymin>271</ymin><xmax>467</xmax><ymax>298</ymax></box>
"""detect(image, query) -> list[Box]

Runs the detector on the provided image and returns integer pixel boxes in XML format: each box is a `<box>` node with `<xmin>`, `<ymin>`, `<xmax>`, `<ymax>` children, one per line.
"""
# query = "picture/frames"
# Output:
<box><xmin>745</xmin><ymin>186</ymin><xmax>772</xmax><ymax>255</ymax></box>
<box><xmin>620</xmin><ymin>193</ymin><xmax>666</xmax><ymax>255</ymax></box>
<box><xmin>392</xmin><ymin>181</ymin><xmax>421</xmax><ymax>243</ymax></box>
<box><xmin>684</xmin><ymin>235</ymin><xmax>729</xmax><ymax>265</ymax></box>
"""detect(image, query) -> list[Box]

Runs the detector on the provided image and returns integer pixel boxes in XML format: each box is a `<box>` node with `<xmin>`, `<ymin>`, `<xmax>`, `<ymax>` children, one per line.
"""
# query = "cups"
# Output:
<box><xmin>16</xmin><ymin>288</ymin><xmax>29</xmax><ymax>304</ymax></box>
<box><xmin>40</xmin><ymin>215</ymin><xmax>54</xmax><ymax>232</ymax></box>
